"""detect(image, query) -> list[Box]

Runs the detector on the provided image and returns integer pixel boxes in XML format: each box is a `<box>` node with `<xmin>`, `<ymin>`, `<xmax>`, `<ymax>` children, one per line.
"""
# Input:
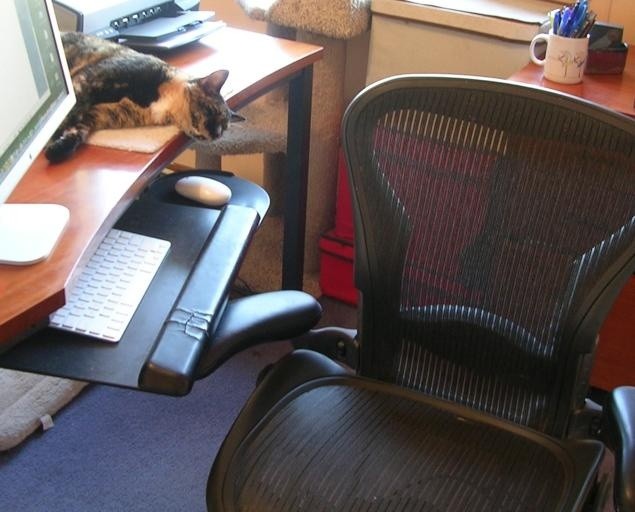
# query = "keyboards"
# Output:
<box><xmin>47</xmin><ymin>228</ymin><xmax>171</xmax><ymax>344</ymax></box>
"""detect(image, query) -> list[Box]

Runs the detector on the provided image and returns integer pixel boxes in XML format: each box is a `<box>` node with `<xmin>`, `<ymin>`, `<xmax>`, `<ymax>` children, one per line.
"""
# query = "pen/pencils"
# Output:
<box><xmin>546</xmin><ymin>0</ymin><xmax>597</xmax><ymax>37</ymax></box>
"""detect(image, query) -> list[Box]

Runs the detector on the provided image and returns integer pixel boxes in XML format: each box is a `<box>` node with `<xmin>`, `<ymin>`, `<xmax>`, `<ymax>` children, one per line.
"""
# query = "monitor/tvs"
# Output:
<box><xmin>0</xmin><ymin>0</ymin><xmax>78</xmax><ymax>267</ymax></box>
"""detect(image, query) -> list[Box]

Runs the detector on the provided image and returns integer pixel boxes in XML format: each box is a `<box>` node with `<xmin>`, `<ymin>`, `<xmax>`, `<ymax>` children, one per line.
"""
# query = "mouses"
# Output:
<box><xmin>175</xmin><ymin>175</ymin><xmax>232</xmax><ymax>208</ymax></box>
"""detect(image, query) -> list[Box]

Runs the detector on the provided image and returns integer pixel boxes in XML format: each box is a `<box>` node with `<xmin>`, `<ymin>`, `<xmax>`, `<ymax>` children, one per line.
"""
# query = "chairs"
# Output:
<box><xmin>188</xmin><ymin>71</ymin><xmax>634</xmax><ymax>511</ymax></box>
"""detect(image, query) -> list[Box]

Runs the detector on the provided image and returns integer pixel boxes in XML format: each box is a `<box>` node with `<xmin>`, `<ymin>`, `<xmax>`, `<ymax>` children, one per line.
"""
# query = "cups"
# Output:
<box><xmin>528</xmin><ymin>29</ymin><xmax>590</xmax><ymax>84</ymax></box>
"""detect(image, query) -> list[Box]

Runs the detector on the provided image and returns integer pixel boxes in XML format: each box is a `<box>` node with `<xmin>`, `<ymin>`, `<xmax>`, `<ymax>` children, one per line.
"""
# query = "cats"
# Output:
<box><xmin>43</xmin><ymin>30</ymin><xmax>248</xmax><ymax>165</ymax></box>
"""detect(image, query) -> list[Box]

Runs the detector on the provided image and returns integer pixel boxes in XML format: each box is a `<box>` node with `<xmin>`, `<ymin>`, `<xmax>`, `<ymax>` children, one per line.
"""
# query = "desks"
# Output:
<box><xmin>1</xmin><ymin>10</ymin><xmax>324</xmax><ymax>357</ymax></box>
